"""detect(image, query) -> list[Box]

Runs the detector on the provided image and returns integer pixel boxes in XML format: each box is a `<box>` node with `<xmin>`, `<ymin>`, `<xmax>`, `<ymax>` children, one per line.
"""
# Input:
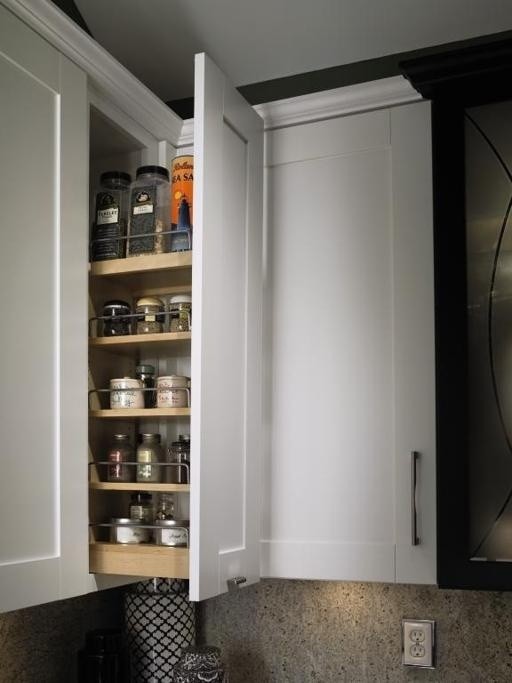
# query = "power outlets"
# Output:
<box><xmin>402</xmin><ymin>619</ymin><xmax>437</xmax><ymax>669</ymax></box>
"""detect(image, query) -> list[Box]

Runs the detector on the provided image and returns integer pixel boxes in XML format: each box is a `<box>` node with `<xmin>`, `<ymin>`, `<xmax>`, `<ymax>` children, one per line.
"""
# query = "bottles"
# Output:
<box><xmin>129</xmin><ymin>493</ymin><xmax>153</xmax><ymax>538</ymax></box>
<box><xmin>77</xmin><ymin>580</ymin><xmax>223</xmax><ymax>682</ymax></box>
<box><xmin>136</xmin><ymin>297</ymin><xmax>163</xmax><ymax>334</ymax></box>
<box><xmin>104</xmin><ymin>301</ymin><xmax>129</xmax><ymax>336</ymax></box>
<box><xmin>167</xmin><ymin>435</ymin><xmax>189</xmax><ymax>483</ymax></box>
<box><xmin>157</xmin><ymin>492</ymin><xmax>179</xmax><ymax>536</ymax></box>
<box><xmin>154</xmin><ymin>514</ymin><xmax>188</xmax><ymax>548</ymax></box>
<box><xmin>169</xmin><ymin>293</ymin><xmax>190</xmax><ymax>331</ymax></box>
<box><xmin>94</xmin><ymin>170</ymin><xmax>128</xmax><ymax>258</ymax></box>
<box><xmin>110</xmin><ymin>375</ymin><xmax>146</xmax><ymax>407</ymax></box>
<box><xmin>136</xmin><ymin>364</ymin><xmax>154</xmax><ymax>407</ymax></box>
<box><xmin>128</xmin><ymin>165</ymin><xmax>169</xmax><ymax>255</ymax></box>
<box><xmin>137</xmin><ymin>433</ymin><xmax>165</xmax><ymax>482</ymax></box>
<box><xmin>170</xmin><ymin>155</ymin><xmax>195</xmax><ymax>251</ymax></box>
<box><xmin>109</xmin><ymin>434</ymin><xmax>135</xmax><ymax>481</ymax></box>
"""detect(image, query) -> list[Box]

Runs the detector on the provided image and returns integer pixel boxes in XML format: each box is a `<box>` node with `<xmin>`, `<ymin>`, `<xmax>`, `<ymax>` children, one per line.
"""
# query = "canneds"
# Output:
<box><xmin>110</xmin><ymin>379</ymin><xmax>144</xmax><ymax>410</ymax></box>
<box><xmin>156</xmin><ymin>520</ymin><xmax>189</xmax><ymax>548</ymax></box>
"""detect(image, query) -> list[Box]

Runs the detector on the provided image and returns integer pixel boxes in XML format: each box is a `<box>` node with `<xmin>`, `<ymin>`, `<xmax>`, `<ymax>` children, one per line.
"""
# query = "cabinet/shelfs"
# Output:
<box><xmin>0</xmin><ymin>1</ymin><xmax>467</xmax><ymax>612</ymax></box>
